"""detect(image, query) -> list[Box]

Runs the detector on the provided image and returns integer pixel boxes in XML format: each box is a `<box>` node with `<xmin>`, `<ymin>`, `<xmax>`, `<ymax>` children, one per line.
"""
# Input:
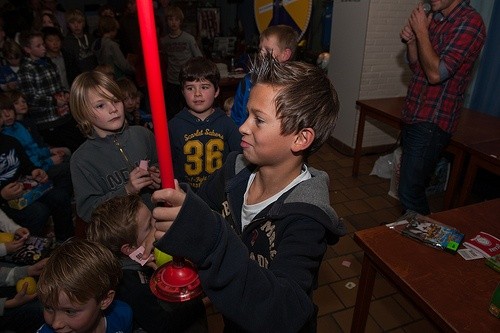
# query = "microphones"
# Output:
<box><xmin>401</xmin><ymin>3</ymin><xmax>432</xmax><ymax>43</ymax></box>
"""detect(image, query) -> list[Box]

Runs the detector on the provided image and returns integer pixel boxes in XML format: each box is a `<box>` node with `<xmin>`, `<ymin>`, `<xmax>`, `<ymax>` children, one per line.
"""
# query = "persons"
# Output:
<box><xmin>393</xmin><ymin>1</ymin><xmax>487</xmax><ymax>218</ymax></box>
<box><xmin>0</xmin><ymin>0</ymin><xmax>343</xmax><ymax>333</ymax></box>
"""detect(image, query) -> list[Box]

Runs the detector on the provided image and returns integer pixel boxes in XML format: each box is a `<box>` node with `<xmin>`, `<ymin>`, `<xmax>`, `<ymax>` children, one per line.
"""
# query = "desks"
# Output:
<box><xmin>352</xmin><ymin>95</ymin><xmax>500</xmax><ymax>210</ymax></box>
<box><xmin>213</xmin><ymin>68</ymin><xmax>246</xmax><ymax>110</ymax></box>
<box><xmin>351</xmin><ymin>197</ymin><xmax>500</xmax><ymax>333</ymax></box>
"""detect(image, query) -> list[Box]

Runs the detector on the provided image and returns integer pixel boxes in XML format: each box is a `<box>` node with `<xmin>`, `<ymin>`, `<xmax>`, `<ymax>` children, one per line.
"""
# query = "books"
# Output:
<box><xmin>394</xmin><ymin>215</ymin><xmax>464</xmax><ymax>251</ymax></box>
